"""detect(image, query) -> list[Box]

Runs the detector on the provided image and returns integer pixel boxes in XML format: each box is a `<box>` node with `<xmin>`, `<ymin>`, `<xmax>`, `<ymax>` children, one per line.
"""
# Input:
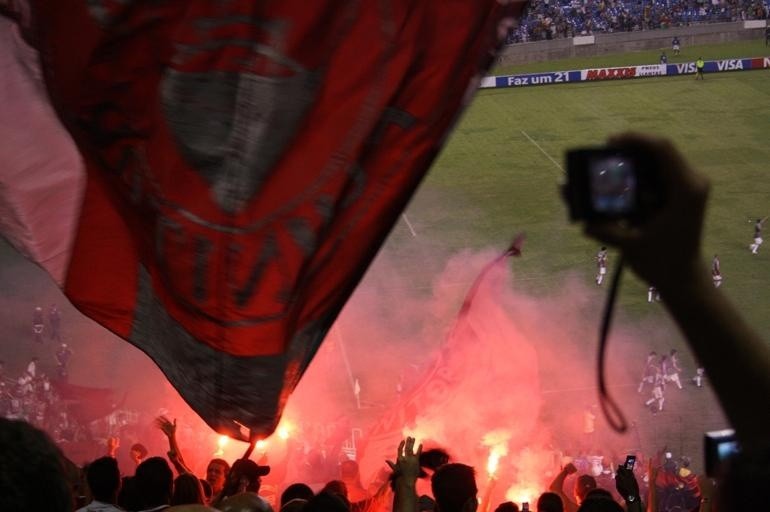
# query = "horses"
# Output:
<box><xmin>624</xmin><ymin>455</ymin><xmax>637</xmax><ymax>473</ymax></box>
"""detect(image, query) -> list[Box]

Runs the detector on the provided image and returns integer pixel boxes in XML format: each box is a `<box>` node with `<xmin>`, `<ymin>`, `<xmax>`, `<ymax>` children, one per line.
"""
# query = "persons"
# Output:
<box><xmin>595</xmin><ymin>247</ymin><xmax>607</xmax><ymax>285</ymax></box>
<box><xmin>563</xmin><ymin>132</ymin><xmax>769</xmax><ymax>510</ymax></box>
<box><xmin>694</xmin><ymin>57</ymin><xmax>705</xmax><ymax>80</ymax></box>
<box><xmin>692</xmin><ymin>365</ymin><xmax>705</xmax><ymax>387</ymax></box>
<box><xmin>712</xmin><ymin>255</ymin><xmax>722</xmax><ymax>288</ymax></box>
<box><xmin>27</xmin><ymin>357</ymin><xmax>41</xmax><ymax>378</ymax></box>
<box><xmin>506</xmin><ymin>0</ymin><xmax>770</xmax><ymax>46</ymax></box>
<box><xmin>0</xmin><ymin>372</ymin><xmax>758</xmax><ymax>512</ymax></box>
<box><xmin>48</xmin><ymin>304</ymin><xmax>61</xmax><ymax>340</ymax></box>
<box><xmin>668</xmin><ymin>349</ymin><xmax>684</xmax><ymax>390</ymax></box>
<box><xmin>660</xmin><ymin>355</ymin><xmax>670</xmax><ymax>383</ymax></box>
<box><xmin>32</xmin><ymin>306</ymin><xmax>45</xmax><ymax>345</ymax></box>
<box><xmin>55</xmin><ymin>342</ymin><xmax>73</xmax><ymax>379</ymax></box>
<box><xmin>672</xmin><ymin>37</ymin><xmax>680</xmax><ymax>55</ymax></box>
<box><xmin>660</xmin><ymin>52</ymin><xmax>667</xmax><ymax>64</ymax></box>
<box><xmin>639</xmin><ymin>352</ymin><xmax>657</xmax><ymax>392</ymax></box>
<box><xmin>643</xmin><ymin>371</ymin><xmax>665</xmax><ymax>410</ymax></box>
<box><xmin>749</xmin><ymin>216</ymin><xmax>768</xmax><ymax>254</ymax></box>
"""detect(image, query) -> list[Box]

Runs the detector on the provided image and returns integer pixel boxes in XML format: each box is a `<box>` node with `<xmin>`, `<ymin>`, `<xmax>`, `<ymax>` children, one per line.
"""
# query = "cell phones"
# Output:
<box><xmin>704</xmin><ymin>427</ymin><xmax>736</xmax><ymax>478</ymax></box>
<box><xmin>564</xmin><ymin>141</ymin><xmax>635</xmax><ymax>223</ymax></box>
